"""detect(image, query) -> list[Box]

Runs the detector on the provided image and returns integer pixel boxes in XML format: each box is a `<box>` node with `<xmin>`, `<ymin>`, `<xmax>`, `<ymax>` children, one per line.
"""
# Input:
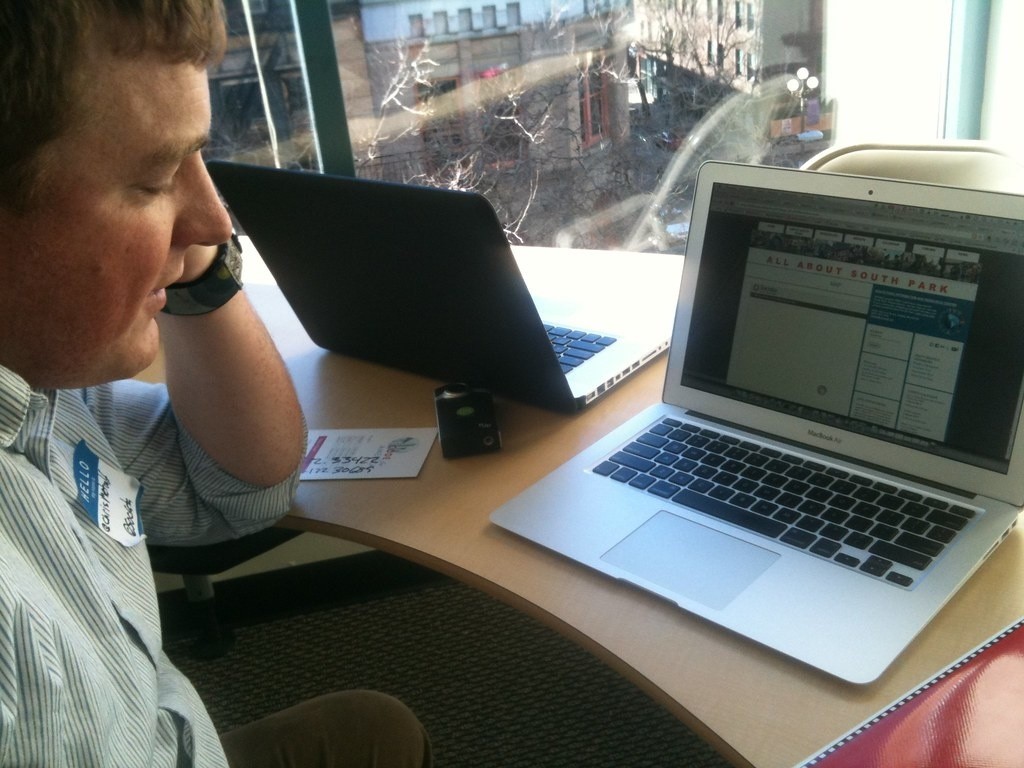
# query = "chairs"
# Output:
<box><xmin>798</xmin><ymin>141</ymin><xmax>1024</xmax><ymax>194</ymax></box>
<box><xmin>147</xmin><ymin>528</ymin><xmax>304</xmax><ymax>662</ymax></box>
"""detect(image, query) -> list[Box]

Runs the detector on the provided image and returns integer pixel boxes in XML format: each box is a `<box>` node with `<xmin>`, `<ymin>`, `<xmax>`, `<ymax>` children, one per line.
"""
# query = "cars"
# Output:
<box><xmin>654</xmin><ymin>130</ymin><xmax>688</xmax><ymax>151</ymax></box>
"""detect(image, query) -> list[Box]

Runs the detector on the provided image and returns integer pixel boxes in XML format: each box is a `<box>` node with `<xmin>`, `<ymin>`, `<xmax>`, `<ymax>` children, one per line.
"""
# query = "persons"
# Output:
<box><xmin>1</xmin><ymin>0</ymin><xmax>432</xmax><ymax>768</ymax></box>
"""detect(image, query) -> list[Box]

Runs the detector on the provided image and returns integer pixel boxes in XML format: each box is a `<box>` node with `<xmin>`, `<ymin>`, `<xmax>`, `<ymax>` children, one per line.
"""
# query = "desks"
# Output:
<box><xmin>132</xmin><ymin>235</ymin><xmax>1024</xmax><ymax>768</ymax></box>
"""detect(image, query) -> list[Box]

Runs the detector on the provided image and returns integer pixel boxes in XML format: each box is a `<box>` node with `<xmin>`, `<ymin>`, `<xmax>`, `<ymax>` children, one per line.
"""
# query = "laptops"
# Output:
<box><xmin>491</xmin><ymin>159</ymin><xmax>1024</xmax><ymax>685</ymax></box>
<box><xmin>204</xmin><ymin>157</ymin><xmax>680</xmax><ymax>411</ymax></box>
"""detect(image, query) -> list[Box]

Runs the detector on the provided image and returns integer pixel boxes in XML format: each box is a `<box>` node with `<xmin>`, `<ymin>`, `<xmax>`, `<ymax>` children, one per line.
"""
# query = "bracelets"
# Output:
<box><xmin>160</xmin><ymin>227</ymin><xmax>243</xmax><ymax>316</ymax></box>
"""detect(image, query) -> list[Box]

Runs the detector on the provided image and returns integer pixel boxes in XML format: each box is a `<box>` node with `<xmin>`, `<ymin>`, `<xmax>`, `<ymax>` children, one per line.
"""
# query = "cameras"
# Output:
<box><xmin>431</xmin><ymin>381</ymin><xmax>504</xmax><ymax>458</ymax></box>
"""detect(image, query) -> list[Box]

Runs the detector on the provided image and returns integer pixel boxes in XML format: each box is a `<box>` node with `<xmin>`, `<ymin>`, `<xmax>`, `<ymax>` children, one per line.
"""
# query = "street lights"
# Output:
<box><xmin>784</xmin><ymin>65</ymin><xmax>820</xmax><ymax>172</ymax></box>
